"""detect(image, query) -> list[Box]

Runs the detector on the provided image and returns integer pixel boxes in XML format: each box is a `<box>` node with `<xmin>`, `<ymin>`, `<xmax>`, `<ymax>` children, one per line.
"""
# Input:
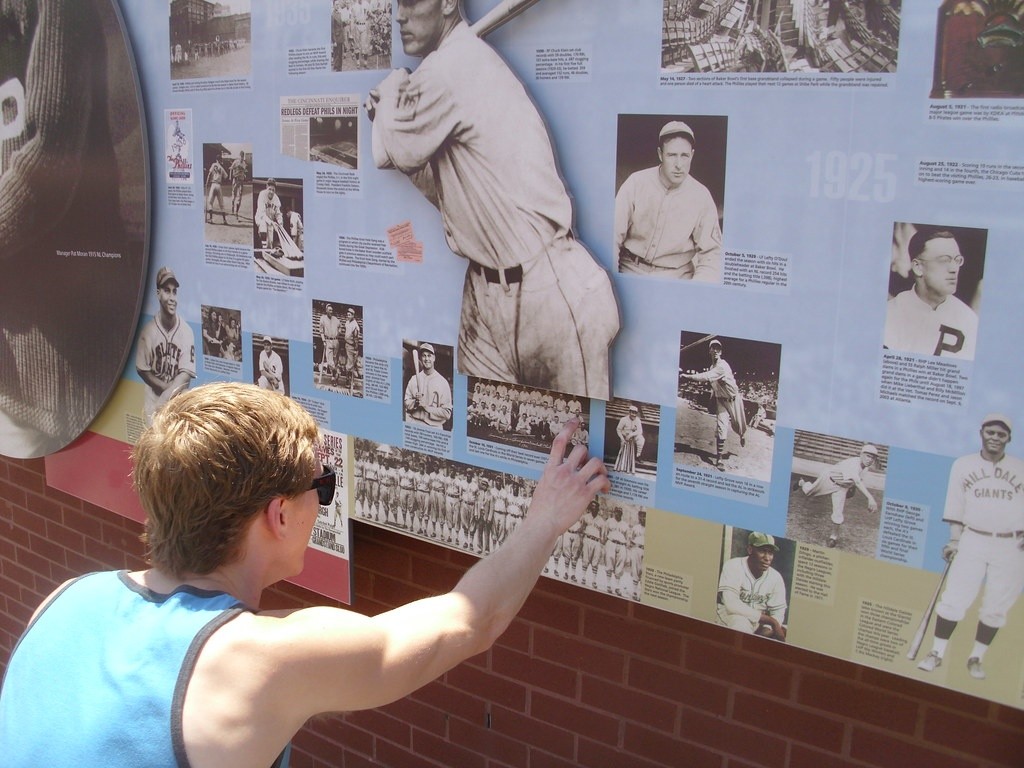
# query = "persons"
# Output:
<box><xmin>0</xmin><ymin>382</ymin><xmax>609</xmax><ymax>768</ymax></box>
<box><xmin>132</xmin><ymin>0</ymin><xmax>1024</xmax><ymax>681</ymax></box>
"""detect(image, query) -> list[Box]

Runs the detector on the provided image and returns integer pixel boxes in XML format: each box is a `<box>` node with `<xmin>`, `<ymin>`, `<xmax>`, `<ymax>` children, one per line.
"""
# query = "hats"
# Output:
<box><xmin>629</xmin><ymin>406</ymin><xmax>639</xmax><ymax>413</ymax></box>
<box><xmin>419</xmin><ymin>343</ymin><xmax>435</xmax><ymax>355</ymax></box>
<box><xmin>658</xmin><ymin>121</ymin><xmax>695</xmax><ymax>145</ymax></box>
<box><xmin>348</xmin><ymin>307</ymin><xmax>356</xmax><ymax>315</ymax></box>
<box><xmin>748</xmin><ymin>532</ymin><xmax>780</xmax><ymax>552</ymax></box>
<box><xmin>982</xmin><ymin>413</ymin><xmax>1012</xmax><ymax>433</ymax></box>
<box><xmin>862</xmin><ymin>445</ymin><xmax>879</xmax><ymax>457</ymax></box>
<box><xmin>708</xmin><ymin>340</ymin><xmax>723</xmax><ymax>348</ymax></box>
<box><xmin>354</xmin><ymin>448</ymin><xmax>647</xmax><ymax>519</ymax></box>
<box><xmin>263</xmin><ymin>336</ymin><xmax>272</xmax><ymax>345</ymax></box>
<box><xmin>267</xmin><ymin>178</ymin><xmax>276</xmax><ymax>187</ymax></box>
<box><xmin>157</xmin><ymin>267</ymin><xmax>180</xmax><ymax>287</ymax></box>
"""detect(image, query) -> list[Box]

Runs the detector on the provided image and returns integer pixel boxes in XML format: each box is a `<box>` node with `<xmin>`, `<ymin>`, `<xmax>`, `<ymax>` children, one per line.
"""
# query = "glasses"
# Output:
<box><xmin>307</xmin><ymin>463</ymin><xmax>336</xmax><ymax>506</ymax></box>
<box><xmin>917</xmin><ymin>256</ymin><xmax>964</xmax><ymax>266</ymax></box>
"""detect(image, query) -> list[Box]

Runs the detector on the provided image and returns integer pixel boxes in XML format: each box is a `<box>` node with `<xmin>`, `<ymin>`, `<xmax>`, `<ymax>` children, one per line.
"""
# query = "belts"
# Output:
<box><xmin>355</xmin><ymin>474</ymin><xmax>645</xmax><ymax>557</ymax></box>
<box><xmin>326</xmin><ymin>337</ymin><xmax>338</xmax><ymax>340</ymax></box>
<box><xmin>968</xmin><ymin>526</ymin><xmax>1022</xmax><ymax>538</ymax></box>
<box><xmin>717</xmin><ymin>397</ymin><xmax>735</xmax><ymax>402</ymax></box>
<box><xmin>623</xmin><ymin>248</ymin><xmax>652</xmax><ymax>266</ymax></box>
<box><xmin>830</xmin><ymin>477</ymin><xmax>847</xmax><ymax>488</ymax></box>
<box><xmin>346</xmin><ymin>342</ymin><xmax>355</xmax><ymax>346</ymax></box>
<box><xmin>469</xmin><ymin>260</ymin><xmax>523</xmax><ymax>284</ymax></box>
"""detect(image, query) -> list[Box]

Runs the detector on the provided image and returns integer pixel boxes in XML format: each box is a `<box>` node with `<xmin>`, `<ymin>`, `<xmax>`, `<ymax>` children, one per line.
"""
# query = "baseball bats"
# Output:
<box><xmin>411</xmin><ymin>349</ymin><xmax>424</xmax><ymax>395</ymax></box>
<box><xmin>612</xmin><ymin>439</ymin><xmax>636</xmax><ymax>473</ymax></box>
<box><xmin>681</xmin><ymin>332</ymin><xmax>718</xmax><ymax>354</ymax></box>
<box><xmin>905</xmin><ymin>551</ymin><xmax>955</xmax><ymax>662</ymax></box>
<box><xmin>271</xmin><ymin>381</ymin><xmax>277</xmax><ymax>392</ymax></box>
<box><xmin>318</xmin><ymin>345</ymin><xmax>325</xmax><ymax>385</ymax></box>
<box><xmin>470</xmin><ymin>0</ymin><xmax>539</xmax><ymax>38</ymax></box>
<box><xmin>275</xmin><ymin>223</ymin><xmax>304</xmax><ymax>260</ymax></box>
<box><xmin>253</xmin><ymin>257</ymin><xmax>271</xmax><ymax>275</ymax></box>
<box><xmin>348</xmin><ymin>366</ymin><xmax>355</xmax><ymax>396</ymax></box>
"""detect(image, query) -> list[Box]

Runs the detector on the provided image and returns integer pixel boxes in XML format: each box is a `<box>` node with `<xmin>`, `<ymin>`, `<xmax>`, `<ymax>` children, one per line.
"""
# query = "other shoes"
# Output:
<box><xmin>827</xmin><ymin>535</ymin><xmax>839</xmax><ymax>548</ymax></box>
<box><xmin>331</xmin><ymin>374</ymin><xmax>339</xmax><ymax>387</ymax></box>
<box><xmin>741</xmin><ymin>437</ymin><xmax>745</xmax><ymax>447</ymax></box>
<box><xmin>967</xmin><ymin>657</ymin><xmax>986</xmax><ymax>680</ymax></box>
<box><xmin>793</xmin><ymin>477</ymin><xmax>803</xmax><ymax>491</ymax></box>
<box><xmin>716</xmin><ymin>462</ymin><xmax>726</xmax><ymax>471</ymax></box>
<box><xmin>354</xmin><ymin>510</ymin><xmax>643</xmax><ymax>603</ymax></box>
<box><xmin>918</xmin><ymin>650</ymin><xmax>943</xmax><ymax>671</ymax></box>
